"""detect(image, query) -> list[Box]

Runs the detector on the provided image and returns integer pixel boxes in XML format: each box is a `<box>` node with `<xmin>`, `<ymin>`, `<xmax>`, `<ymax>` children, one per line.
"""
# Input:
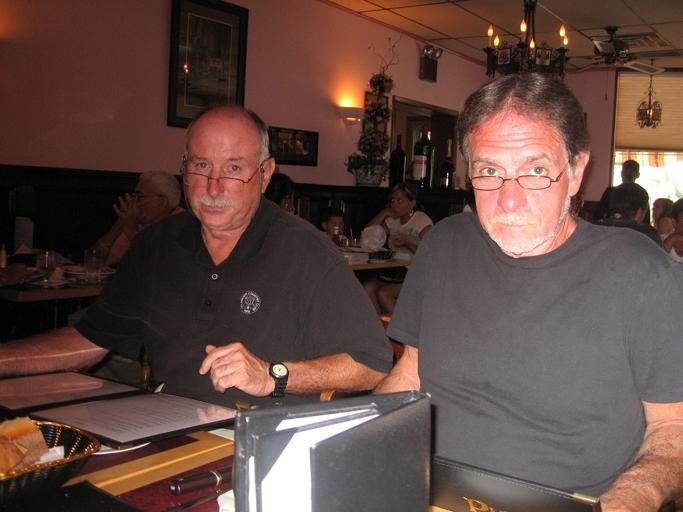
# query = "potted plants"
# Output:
<box><xmin>345</xmin><ymin>70</ymin><xmax>394</xmax><ymax>187</ymax></box>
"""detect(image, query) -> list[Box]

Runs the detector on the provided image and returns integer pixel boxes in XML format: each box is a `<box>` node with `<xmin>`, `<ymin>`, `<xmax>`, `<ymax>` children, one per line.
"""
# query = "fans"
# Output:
<box><xmin>568</xmin><ymin>29</ymin><xmax>667</xmax><ymax>77</ymax></box>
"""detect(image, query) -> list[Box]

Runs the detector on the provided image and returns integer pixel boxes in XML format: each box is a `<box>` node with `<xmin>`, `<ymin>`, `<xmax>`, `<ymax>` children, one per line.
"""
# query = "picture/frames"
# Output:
<box><xmin>266</xmin><ymin>124</ymin><xmax>320</xmax><ymax>168</ymax></box>
<box><xmin>164</xmin><ymin>0</ymin><xmax>251</xmax><ymax>132</ymax></box>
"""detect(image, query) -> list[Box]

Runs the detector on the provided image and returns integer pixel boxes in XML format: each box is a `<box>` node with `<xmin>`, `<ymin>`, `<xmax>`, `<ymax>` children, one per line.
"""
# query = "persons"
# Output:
<box><xmin>592</xmin><ymin>159</ymin><xmax>651</xmax><ymax>223</ymax></box>
<box><xmin>588</xmin><ymin>182</ymin><xmax>663</xmax><ymax>248</ymax></box>
<box><xmin>658</xmin><ymin>195</ymin><xmax>683</xmax><ymax>261</ymax></box>
<box><xmin>263</xmin><ymin>173</ymin><xmax>295</xmax><ymax>218</ymax></box>
<box><xmin>88</xmin><ymin>167</ymin><xmax>182</xmax><ymax>268</ymax></box>
<box><xmin>369</xmin><ymin>73</ymin><xmax>683</xmax><ymax>511</ymax></box>
<box><xmin>0</xmin><ymin>105</ymin><xmax>394</xmax><ymax>411</ymax></box>
<box><xmin>321</xmin><ymin>208</ymin><xmax>344</xmax><ymax>243</ymax></box>
<box><xmin>363</xmin><ymin>180</ymin><xmax>433</xmax><ymax>316</ymax></box>
<box><xmin>650</xmin><ymin>197</ymin><xmax>673</xmax><ymax>247</ymax></box>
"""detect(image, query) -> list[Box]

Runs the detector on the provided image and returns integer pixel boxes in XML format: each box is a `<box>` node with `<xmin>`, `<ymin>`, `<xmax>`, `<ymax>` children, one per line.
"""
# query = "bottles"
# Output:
<box><xmin>333</xmin><ymin>226</ymin><xmax>341</xmax><ymax>248</ymax></box>
<box><xmin>390</xmin><ymin>135</ymin><xmax>407</xmax><ymax>185</ymax></box>
<box><xmin>413</xmin><ymin>126</ymin><xmax>435</xmax><ymax>190</ymax></box>
<box><xmin>439</xmin><ymin>140</ymin><xmax>456</xmax><ymax>191</ymax></box>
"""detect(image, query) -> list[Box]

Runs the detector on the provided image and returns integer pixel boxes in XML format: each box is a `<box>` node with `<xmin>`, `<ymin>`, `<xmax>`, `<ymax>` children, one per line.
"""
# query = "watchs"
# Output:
<box><xmin>268</xmin><ymin>362</ymin><xmax>288</xmax><ymax>395</ymax></box>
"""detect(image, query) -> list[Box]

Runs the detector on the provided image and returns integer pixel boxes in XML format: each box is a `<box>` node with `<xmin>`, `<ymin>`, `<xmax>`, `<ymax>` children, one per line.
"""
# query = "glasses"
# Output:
<box><xmin>131</xmin><ymin>192</ymin><xmax>166</xmax><ymax>201</ymax></box>
<box><xmin>179</xmin><ymin>154</ymin><xmax>271</xmax><ymax>193</ymax></box>
<box><xmin>465</xmin><ymin>154</ymin><xmax>573</xmax><ymax>191</ymax></box>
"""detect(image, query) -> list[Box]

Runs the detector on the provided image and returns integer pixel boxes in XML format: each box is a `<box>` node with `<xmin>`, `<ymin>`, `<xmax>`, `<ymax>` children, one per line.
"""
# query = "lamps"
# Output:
<box><xmin>637</xmin><ymin>88</ymin><xmax>662</xmax><ymax>131</ymax></box>
<box><xmin>419</xmin><ymin>45</ymin><xmax>442</xmax><ymax>83</ymax></box>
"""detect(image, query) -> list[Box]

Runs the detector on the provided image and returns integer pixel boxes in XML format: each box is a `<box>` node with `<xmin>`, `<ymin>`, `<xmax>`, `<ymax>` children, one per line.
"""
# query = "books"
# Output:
<box><xmin>0</xmin><ymin>369</ymin><xmax>260</xmax><ymax>450</ymax></box>
<box><xmin>232</xmin><ymin>390</ymin><xmax>431</xmax><ymax>511</ymax></box>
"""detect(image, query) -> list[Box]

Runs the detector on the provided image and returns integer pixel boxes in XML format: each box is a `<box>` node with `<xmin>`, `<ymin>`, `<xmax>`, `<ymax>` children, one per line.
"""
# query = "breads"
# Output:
<box><xmin>0</xmin><ymin>417</ymin><xmax>50</xmax><ymax>478</ymax></box>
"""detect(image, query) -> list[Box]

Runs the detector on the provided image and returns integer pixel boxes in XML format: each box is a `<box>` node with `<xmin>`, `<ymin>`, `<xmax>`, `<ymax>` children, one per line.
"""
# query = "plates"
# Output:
<box><xmin>64</xmin><ymin>268</ymin><xmax>116</xmax><ymax>276</ymax></box>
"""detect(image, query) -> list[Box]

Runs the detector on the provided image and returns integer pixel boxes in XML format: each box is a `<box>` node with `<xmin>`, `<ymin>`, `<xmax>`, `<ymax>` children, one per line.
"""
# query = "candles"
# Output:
<box><xmin>491</xmin><ymin>31</ymin><xmax>499</xmax><ymax>55</ymax></box>
<box><xmin>529</xmin><ymin>38</ymin><xmax>538</xmax><ymax>64</ymax></box>
<box><xmin>486</xmin><ymin>23</ymin><xmax>496</xmax><ymax>50</ymax></box>
<box><xmin>519</xmin><ymin>21</ymin><xmax>527</xmax><ymax>45</ymax></box>
<box><xmin>563</xmin><ymin>33</ymin><xmax>571</xmax><ymax>53</ymax></box>
<box><xmin>559</xmin><ymin>24</ymin><xmax>566</xmax><ymax>46</ymax></box>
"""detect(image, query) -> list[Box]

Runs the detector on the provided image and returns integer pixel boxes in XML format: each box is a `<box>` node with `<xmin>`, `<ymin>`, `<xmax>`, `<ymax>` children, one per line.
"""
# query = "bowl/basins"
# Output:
<box><xmin>0</xmin><ymin>422</ymin><xmax>101</xmax><ymax>497</ymax></box>
<box><xmin>370</xmin><ymin>250</ymin><xmax>393</xmax><ymax>260</ymax></box>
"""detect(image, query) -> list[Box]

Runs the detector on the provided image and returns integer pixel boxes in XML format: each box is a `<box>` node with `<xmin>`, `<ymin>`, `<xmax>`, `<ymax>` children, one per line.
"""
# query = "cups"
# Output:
<box><xmin>36</xmin><ymin>250</ymin><xmax>57</xmax><ymax>289</ymax></box>
<box><xmin>83</xmin><ymin>243</ymin><xmax>104</xmax><ymax>284</ymax></box>
<box><xmin>350</xmin><ymin>238</ymin><xmax>359</xmax><ymax>247</ymax></box>
